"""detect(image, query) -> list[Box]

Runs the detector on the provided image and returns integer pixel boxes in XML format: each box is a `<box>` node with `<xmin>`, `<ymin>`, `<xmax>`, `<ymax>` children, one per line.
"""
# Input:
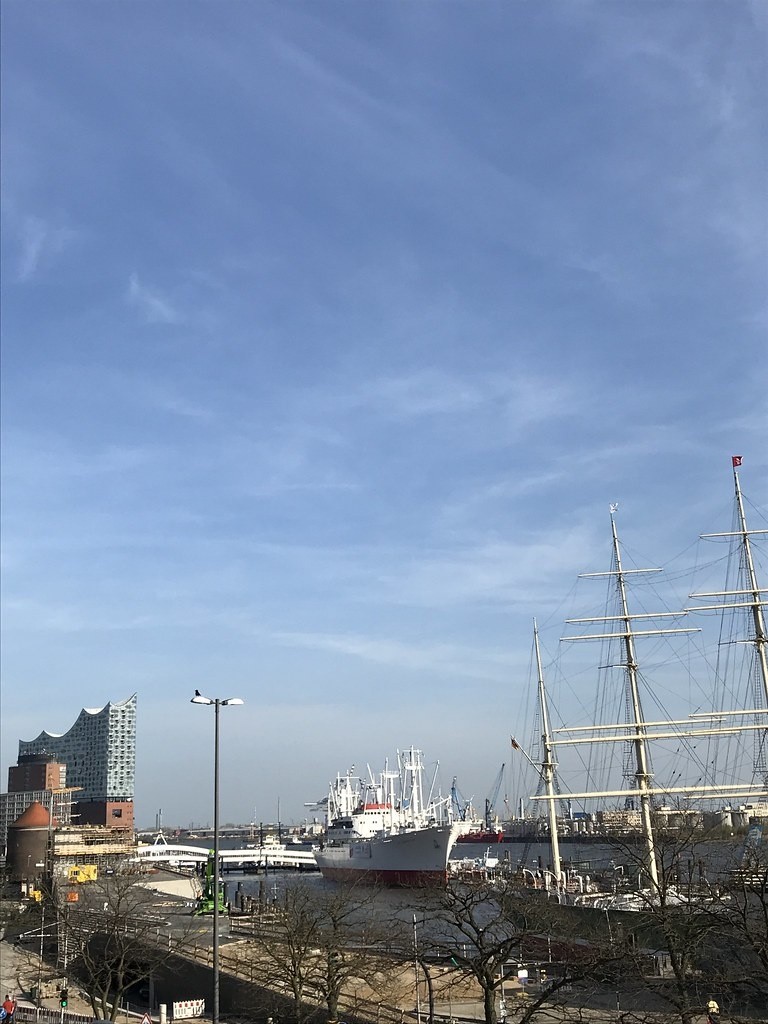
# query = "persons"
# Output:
<box><xmin>1</xmin><ymin>995</ymin><xmax>17</xmax><ymax>1024</ymax></box>
<box><xmin>18</xmin><ymin>901</ymin><xmax>27</xmax><ymax>913</ymax></box>
<box><xmin>706</xmin><ymin>994</ymin><xmax>721</xmax><ymax>1024</ymax></box>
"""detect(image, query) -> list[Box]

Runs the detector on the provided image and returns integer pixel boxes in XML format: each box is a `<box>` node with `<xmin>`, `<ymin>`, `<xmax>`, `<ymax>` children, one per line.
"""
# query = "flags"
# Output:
<box><xmin>733</xmin><ymin>456</ymin><xmax>743</xmax><ymax>467</ymax></box>
<box><xmin>511</xmin><ymin>740</ymin><xmax>519</xmax><ymax>750</ymax></box>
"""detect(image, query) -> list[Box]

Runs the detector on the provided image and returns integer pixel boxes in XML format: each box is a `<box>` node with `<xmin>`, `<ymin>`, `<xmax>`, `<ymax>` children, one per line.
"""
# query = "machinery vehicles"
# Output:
<box><xmin>191</xmin><ymin>848</ymin><xmax>231</xmax><ymax>917</ymax></box>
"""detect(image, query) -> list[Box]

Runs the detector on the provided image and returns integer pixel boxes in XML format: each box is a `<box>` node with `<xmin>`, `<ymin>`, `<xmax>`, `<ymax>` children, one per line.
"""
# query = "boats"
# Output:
<box><xmin>305</xmin><ymin>744</ymin><xmax>470</xmax><ymax>888</ymax></box>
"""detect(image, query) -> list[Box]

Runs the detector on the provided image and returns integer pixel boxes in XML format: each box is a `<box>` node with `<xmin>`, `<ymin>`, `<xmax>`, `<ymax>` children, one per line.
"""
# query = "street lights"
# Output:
<box><xmin>191</xmin><ymin>695</ymin><xmax>243</xmax><ymax>1023</ymax></box>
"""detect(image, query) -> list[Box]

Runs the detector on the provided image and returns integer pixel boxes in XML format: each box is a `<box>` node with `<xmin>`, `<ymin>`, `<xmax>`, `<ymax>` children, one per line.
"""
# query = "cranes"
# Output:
<box><xmin>519</xmin><ymin>681</ymin><xmax>589</xmax><ymax>873</ymax></box>
<box><xmin>448</xmin><ymin>775</ymin><xmax>474</xmax><ymax>822</ymax></box>
<box><xmin>482</xmin><ymin>762</ymin><xmax>506</xmax><ymax>828</ymax></box>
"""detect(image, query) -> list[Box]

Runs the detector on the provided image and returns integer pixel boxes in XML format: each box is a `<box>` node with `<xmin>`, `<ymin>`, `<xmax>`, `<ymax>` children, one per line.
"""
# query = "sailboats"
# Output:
<box><xmin>522</xmin><ymin>455</ymin><xmax>768</xmax><ymax>917</ymax></box>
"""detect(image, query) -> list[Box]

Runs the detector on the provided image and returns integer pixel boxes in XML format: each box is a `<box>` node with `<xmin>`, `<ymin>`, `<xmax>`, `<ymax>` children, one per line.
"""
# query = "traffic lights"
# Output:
<box><xmin>59</xmin><ymin>988</ymin><xmax>69</xmax><ymax>1007</ymax></box>
